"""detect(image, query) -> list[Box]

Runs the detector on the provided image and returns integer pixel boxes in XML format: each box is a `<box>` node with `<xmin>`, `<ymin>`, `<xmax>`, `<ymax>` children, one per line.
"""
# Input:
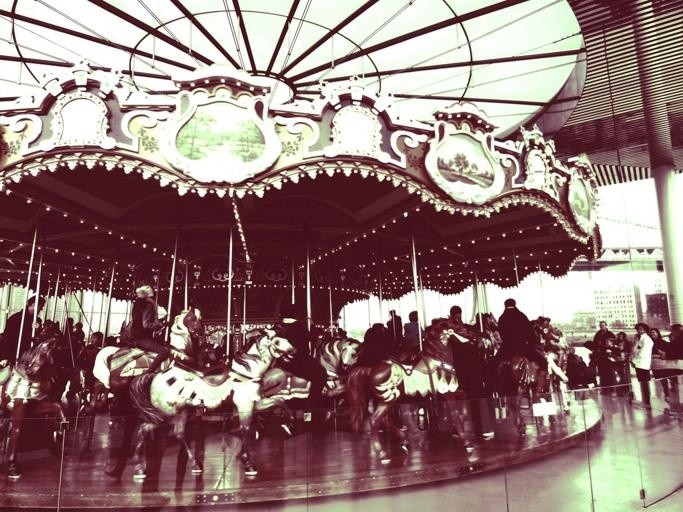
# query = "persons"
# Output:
<box><xmin>562</xmin><ymin>321</ymin><xmax>682</xmax><ymax>413</ymax></box>
<box><xmin>1</xmin><ymin>285</ymin><xmax>562</xmax><ymax>456</ymax></box>
